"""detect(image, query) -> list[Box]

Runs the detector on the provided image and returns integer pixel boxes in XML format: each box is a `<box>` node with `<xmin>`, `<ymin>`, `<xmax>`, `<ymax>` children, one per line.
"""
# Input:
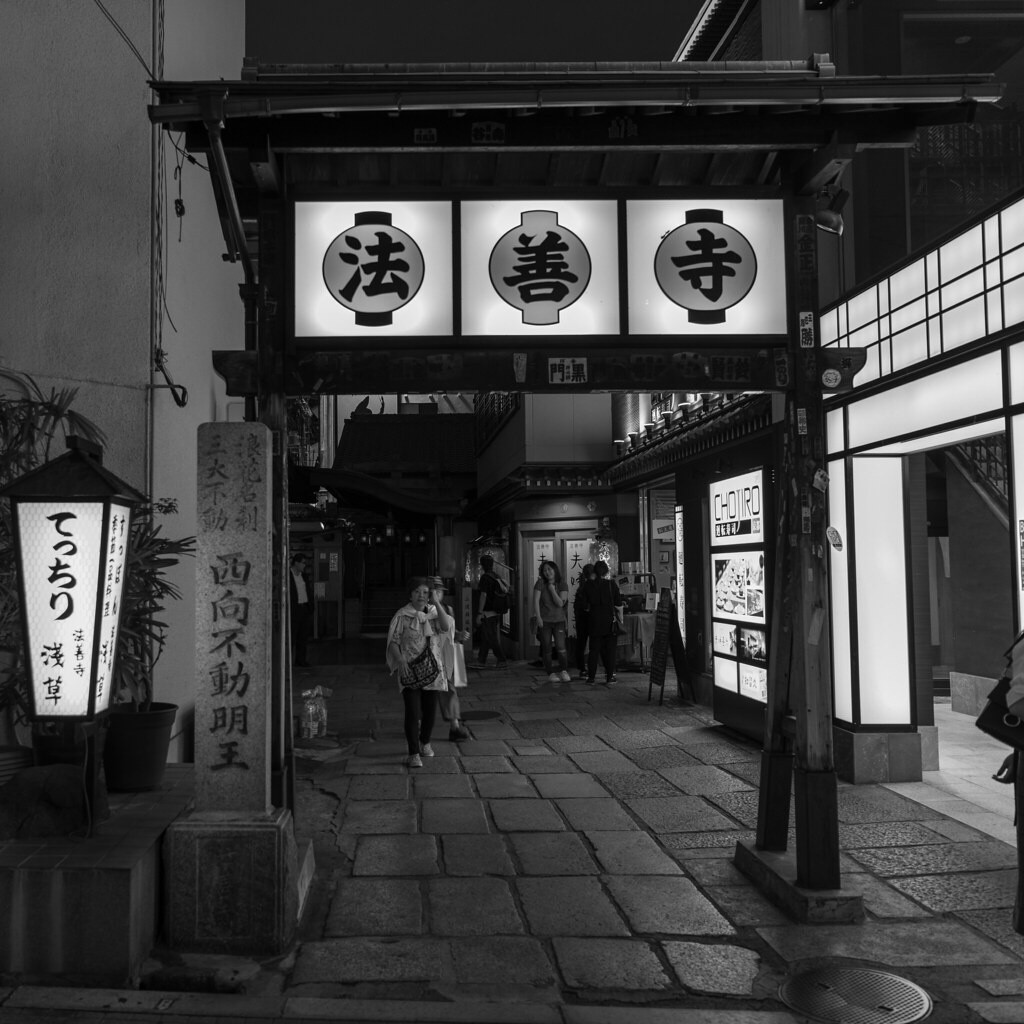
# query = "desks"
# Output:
<box><xmin>617</xmin><ymin>613</ymin><xmax>655</xmax><ymax>673</ymax></box>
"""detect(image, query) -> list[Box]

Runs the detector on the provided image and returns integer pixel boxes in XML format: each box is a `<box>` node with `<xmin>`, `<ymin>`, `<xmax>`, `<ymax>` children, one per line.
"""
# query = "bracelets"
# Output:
<box><xmin>477</xmin><ymin>612</ymin><xmax>482</xmax><ymax>615</ymax></box>
<box><xmin>551</xmin><ymin>590</ymin><xmax>555</xmax><ymax>592</ymax></box>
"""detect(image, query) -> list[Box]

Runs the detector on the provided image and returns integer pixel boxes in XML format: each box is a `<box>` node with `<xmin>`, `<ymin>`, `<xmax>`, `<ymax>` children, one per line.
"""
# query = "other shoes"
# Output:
<box><xmin>549</xmin><ymin>673</ymin><xmax>561</xmax><ymax>682</ymax></box>
<box><xmin>528</xmin><ymin>659</ymin><xmax>545</xmax><ymax>668</ymax></box>
<box><xmin>560</xmin><ymin>670</ymin><xmax>570</xmax><ymax>682</ymax></box>
<box><xmin>468</xmin><ymin>661</ymin><xmax>487</xmax><ymax>669</ymax></box>
<box><xmin>492</xmin><ymin>661</ymin><xmax>509</xmax><ymax>668</ymax></box>
<box><xmin>580</xmin><ymin>671</ymin><xmax>587</xmax><ymax>679</ymax></box>
<box><xmin>449</xmin><ymin>726</ymin><xmax>472</xmax><ymax>741</ymax></box>
<box><xmin>607</xmin><ymin>678</ymin><xmax>617</xmax><ymax>684</ymax></box>
<box><xmin>295</xmin><ymin>661</ymin><xmax>311</xmax><ymax>667</ymax></box>
<box><xmin>585</xmin><ymin>678</ymin><xmax>594</xmax><ymax>684</ymax></box>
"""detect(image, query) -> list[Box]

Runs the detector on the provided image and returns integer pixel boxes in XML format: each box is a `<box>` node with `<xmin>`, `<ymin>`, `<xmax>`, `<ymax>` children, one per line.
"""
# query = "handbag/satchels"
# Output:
<box><xmin>398</xmin><ymin>646</ymin><xmax>440</xmax><ymax>690</ymax></box>
<box><xmin>611</xmin><ymin>616</ymin><xmax>628</xmax><ymax>635</ymax></box>
<box><xmin>452</xmin><ymin>640</ymin><xmax>467</xmax><ymax>687</ymax></box>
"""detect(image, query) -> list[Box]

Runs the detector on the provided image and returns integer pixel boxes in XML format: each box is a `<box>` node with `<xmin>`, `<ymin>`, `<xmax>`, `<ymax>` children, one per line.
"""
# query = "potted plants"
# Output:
<box><xmin>0</xmin><ymin>364</ymin><xmax>196</xmax><ymax>792</ymax></box>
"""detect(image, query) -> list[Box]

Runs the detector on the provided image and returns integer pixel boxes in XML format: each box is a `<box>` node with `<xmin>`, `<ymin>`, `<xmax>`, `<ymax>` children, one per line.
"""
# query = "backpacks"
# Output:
<box><xmin>483</xmin><ymin>573</ymin><xmax>511</xmax><ymax>614</ymax></box>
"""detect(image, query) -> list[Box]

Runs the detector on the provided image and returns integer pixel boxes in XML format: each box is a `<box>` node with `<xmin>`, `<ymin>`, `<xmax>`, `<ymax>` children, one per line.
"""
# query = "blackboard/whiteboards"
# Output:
<box><xmin>649</xmin><ymin>587</ymin><xmax>697</xmax><ymax>687</ymax></box>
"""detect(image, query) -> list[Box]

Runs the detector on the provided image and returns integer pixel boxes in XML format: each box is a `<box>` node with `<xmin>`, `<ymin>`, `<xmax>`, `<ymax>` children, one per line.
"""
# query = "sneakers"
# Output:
<box><xmin>408</xmin><ymin>753</ymin><xmax>422</xmax><ymax>767</ymax></box>
<box><xmin>420</xmin><ymin>742</ymin><xmax>434</xmax><ymax>757</ymax></box>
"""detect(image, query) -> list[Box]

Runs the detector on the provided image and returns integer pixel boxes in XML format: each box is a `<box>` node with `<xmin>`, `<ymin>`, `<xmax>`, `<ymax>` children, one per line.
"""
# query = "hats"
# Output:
<box><xmin>427</xmin><ymin>576</ymin><xmax>448</xmax><ymax>591</ymax></box>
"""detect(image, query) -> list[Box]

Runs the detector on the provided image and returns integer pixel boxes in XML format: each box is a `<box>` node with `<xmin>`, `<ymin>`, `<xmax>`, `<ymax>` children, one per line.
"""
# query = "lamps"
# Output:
<box><xmin>629</xmin><ymin>433</ymin><xmax>636</xmax><ymax>448</ymax></box>
<box><xmin>814</xmin><ymin>189</ymin><xmax>850</xmax><ymax>237</ymax></box>
<box><xmin>678</xmin><ymin>402</ymin><xmax>690</xmax><ymax>422</ymax></box>
<box><xmin>661</xmin><ymin>411</ymin><xmax>672</xmax><ymax>429</ymax></box>
<box><xmin>701</xmin><ymin>394</ymin><xmax>711</xmax><ymax>412</ymax></box>
<box><xmin>613</xmin><ymin>439</ymin><xmax>625</xmax><ymax>457</ymax></box>
<box><xmin>726</xmin><ymin>394</ymin><xmax>734</xmax><ymax>401</ymax></box>
<box><xmin>644</xmin><ymin>423</ymin><xmax>655</xmax><ymax>440</ymax></box>
<box><xmin>0</xmin><ymin>434</ymin><xmax>151</xmax><ymax>824</ymax></box>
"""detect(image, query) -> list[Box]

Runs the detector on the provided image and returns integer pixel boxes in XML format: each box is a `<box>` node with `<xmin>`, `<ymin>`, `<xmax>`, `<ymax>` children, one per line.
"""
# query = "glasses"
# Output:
<box><xmin>300</xmin><ymin>561</ymin><xmax>306</xmax><ymax>564</ymax></box>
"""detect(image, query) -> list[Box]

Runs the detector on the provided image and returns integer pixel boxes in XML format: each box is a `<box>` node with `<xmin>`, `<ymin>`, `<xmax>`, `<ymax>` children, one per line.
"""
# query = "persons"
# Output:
<box><xmin>385</xmin><ymin>576</ymin><xmax>470</xmax><ymax>767</ymax></box>
<box><xmin>290</xmin><ymin>553</ymin><xmax>314</xmax><ymax>667</ymax></box>
<box><xmin>573</xmin><ymin>561</ymin><xmax>624</xmax><ymax>684</ymax></box>
<box><xmin>532</xmin><ymin>561</ymin><xmax>570</xmax><ymax>682</ymax></box>
<box><xmin>467</xmin><ymin>555</ymin><xmax>511</xmax><ymax>670</ymax></box>
<box><xmin>992</xmin><ymin>629</ymin><xmax>1024</xmax><ymax>937</ymax></box>
